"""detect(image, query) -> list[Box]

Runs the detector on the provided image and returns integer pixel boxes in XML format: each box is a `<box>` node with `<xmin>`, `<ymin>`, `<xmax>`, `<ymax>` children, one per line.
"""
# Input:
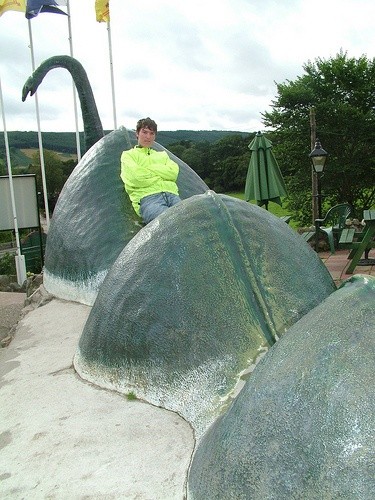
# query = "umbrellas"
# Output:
<box><xmin>244</xmin><ymin>130</ymin><xmax>290</xmax><ymax>211</ymax></box>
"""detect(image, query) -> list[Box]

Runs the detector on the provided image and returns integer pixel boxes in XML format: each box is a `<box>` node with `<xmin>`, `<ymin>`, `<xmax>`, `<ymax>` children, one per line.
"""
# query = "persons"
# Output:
<box><xmin>120</xmin><ymin>117</ymin><xmax>182</xmax><ymax>223</ymax></box>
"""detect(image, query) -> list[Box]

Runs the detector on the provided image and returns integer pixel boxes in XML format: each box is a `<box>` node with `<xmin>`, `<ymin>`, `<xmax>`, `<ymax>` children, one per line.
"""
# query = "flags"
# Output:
<box><xmin>95</xmin><ymin>0</ymin><xmax>110</xmax><ymax>22</ymax></box>
<box><xmin>24</xmin><ymin>1</ymin><xmax>70</xmax><ymax>20</ymax></box>
<box><xmin>1</xmin><ymin>0</ymin><xmax>26</xmax><ymax>18</ymax></box>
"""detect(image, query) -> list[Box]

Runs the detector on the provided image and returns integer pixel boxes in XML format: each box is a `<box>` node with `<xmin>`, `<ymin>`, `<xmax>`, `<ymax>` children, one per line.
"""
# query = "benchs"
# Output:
<box><xmin>301</xmin><ymin>232</ymin><xmax>316</xmax><ymax>245</ymax></box>
<box><xmin>339</xmin><ymin>228</ymin><xmax>356</xmax><ymax>245</ymax></box>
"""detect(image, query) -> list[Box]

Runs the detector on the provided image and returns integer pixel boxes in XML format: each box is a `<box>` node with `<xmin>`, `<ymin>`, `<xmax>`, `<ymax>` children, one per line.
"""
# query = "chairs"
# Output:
<box><xmin>316</xmin><ymin>202</ymin><xmax>353</xmax><ymax>254</ymax></box>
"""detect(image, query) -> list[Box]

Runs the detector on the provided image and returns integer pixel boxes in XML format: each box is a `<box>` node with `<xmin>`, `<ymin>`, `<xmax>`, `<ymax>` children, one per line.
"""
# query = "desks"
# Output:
<box><xmin>280</xmin><ymin>216</ymin><xmax>291</xmax><ymax>223</ymax></box>
<box><xmin>346</xmin><ymin>210</ymin><xmax>375</xmax><ymax>274</ymax></box>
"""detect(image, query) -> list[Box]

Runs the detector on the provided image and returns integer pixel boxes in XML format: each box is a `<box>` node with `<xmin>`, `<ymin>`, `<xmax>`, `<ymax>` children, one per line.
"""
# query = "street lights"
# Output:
<box><xmin>310</xmin><ymin>138</ymin><xmax>328</xmax><ymax>217</ymax></box>
